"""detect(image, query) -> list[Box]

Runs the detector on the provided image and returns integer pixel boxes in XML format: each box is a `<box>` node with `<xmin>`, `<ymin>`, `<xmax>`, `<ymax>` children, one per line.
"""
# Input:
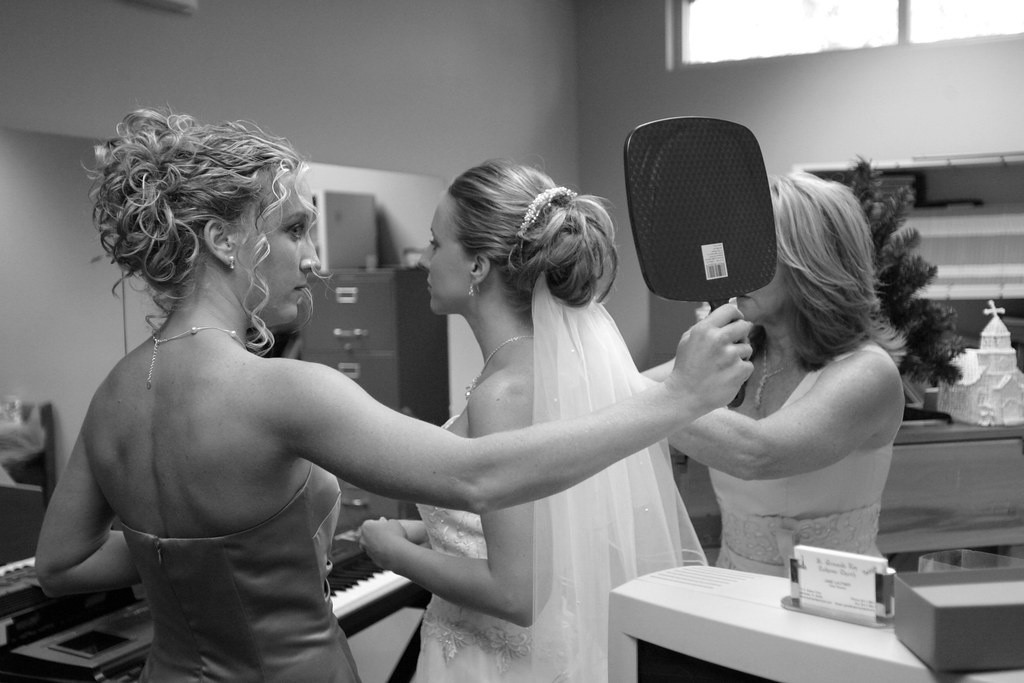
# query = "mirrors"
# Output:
<box><xmin>626</xmin><ymin>116</ymin><xmax>778</xmax><ymax>407</ymax></box>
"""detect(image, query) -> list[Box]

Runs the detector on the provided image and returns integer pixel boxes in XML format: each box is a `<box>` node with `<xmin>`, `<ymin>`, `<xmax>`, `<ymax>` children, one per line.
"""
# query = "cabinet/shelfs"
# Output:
<box><xmin>302</xmin><ymin>266</ymin><xmax>450</xmax><ymax>533</ymax></box>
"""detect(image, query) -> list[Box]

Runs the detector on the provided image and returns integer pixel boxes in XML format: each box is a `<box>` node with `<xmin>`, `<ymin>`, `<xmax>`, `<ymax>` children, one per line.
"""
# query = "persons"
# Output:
<box><xmin>360</xmin><ymin>160</ymin><xmax>640</xmax><ymax>682</ymax></box>
<box><xmin>634</xmin><ymin>172</ymin><xmax>904</xmax><ymax>584</ymax></box>
<box><xmin>36</xmin><ymin>107</ymin><xmax>753</xmax><ymax>682</ymax></box>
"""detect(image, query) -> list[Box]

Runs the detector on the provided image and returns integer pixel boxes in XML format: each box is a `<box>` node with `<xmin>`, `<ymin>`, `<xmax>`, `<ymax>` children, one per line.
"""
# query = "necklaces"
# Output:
<box><xmin>465</xmin><ymin>336</ymin><xmax>536</xmax><ymax>398</ymax></box>
<box><xmin>146</xmin><ymin>327</ymin><xmax>246</xmax><ymax>392</ymax></box>
<box><xmin>756</xmin><ymin>346</ymin><xmax>783</xmax><ymax>408</ymax></box>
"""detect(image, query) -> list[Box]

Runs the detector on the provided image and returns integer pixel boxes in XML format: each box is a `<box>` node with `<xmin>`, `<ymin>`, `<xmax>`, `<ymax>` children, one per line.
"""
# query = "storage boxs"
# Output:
<box><xmin>307</xmin><ymin>192</ymin><xmax>379</xmax><ymax>275</ymax></box>
<box><xmin>895</xmin><ymin>567</ymin><xmax>1024</xmax><ymax>674</ymax></box>
